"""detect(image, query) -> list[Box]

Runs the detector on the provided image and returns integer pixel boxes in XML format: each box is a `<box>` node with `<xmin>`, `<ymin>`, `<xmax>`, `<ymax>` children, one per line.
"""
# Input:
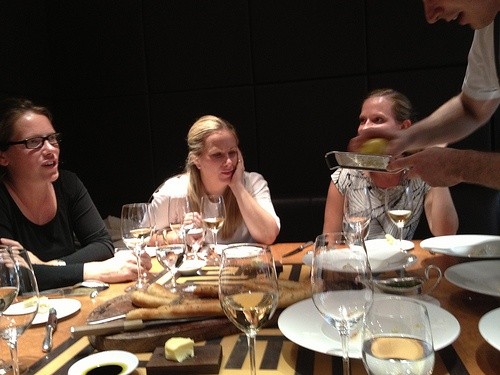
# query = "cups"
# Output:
<box><xmin>362</xmin><ymin>299</ymin><xmax>435</xmax><ymax>375</ymax></box>
<box><xmin>342</xmin><ymin>218</ymin><xmax>370</xmax><ymax>246</ymax></box>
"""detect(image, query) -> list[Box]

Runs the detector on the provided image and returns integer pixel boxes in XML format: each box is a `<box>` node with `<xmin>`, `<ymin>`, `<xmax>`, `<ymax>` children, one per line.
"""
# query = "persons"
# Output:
<box><xmin>349</xmin><ymin>0</ymin><xmax>500</xmax><ymax>189</ymax></box>
<box><xmin>321</xmin><ymin>90</ymin><xmax>458</xmax><ymax>242</ymax></box>
<box><xmin>1</xmin><ymin>97</ymin><xmax>152</xmax><ymax>293</ymax></box>
<box><xmin>141</xmin><ymin>115</ymin><xmax>281</xmax><ymax>247</ymax></box>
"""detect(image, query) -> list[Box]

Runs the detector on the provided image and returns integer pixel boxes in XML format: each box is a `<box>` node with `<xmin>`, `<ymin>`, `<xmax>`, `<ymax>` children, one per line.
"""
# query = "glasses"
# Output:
<box><xmin>9</xmin><ymin>132</ymin><xmax>63</xmax><ymax>150</ymax></box>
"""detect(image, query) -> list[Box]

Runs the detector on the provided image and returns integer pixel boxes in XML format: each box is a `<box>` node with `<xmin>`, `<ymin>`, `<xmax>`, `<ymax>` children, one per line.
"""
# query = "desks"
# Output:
<box><xmin>0</xmin><ymin>242</ymin><xmax>500</xmax><ymax>375</ymax></box>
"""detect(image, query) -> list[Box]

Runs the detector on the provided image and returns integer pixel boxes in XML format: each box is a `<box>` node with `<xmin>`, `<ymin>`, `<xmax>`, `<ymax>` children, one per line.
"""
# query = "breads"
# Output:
<box><xmin>126</xmin><ymin>280</ymin><xmax>313</xmax><ymax>319</ymax></box>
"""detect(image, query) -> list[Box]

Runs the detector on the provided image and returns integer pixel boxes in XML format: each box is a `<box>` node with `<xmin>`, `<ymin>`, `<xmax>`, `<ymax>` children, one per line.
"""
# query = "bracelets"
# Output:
<box><xmin>162</xmin><ymin>230</ymin><xmax>169</xmax><ymax>244</ymax></box>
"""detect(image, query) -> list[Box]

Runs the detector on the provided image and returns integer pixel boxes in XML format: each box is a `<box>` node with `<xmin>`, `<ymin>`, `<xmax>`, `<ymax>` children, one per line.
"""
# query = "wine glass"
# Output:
<box><xmin>119</xmin><ymin>194</ymin><xmax>227</xmax><ymax>292</ymax></box>
<box><xmin>218</xmin><ymin>244</ymin><xmax>279</xmax><ymax>375</ymax></box>
<box><xmin>0</xmin><ymin>244</ymin><xmax>19</xmax><ymax>375</ymax></box>
<box><xmin>385</xmin><ymin>184</ymin><xmax>412</xmax><ymax>243</ymax></box>
<box><xmin>0</xmin><ymin>248</ymin><xmax>40</xmax><ymax>375</ymax></box>
<box><xmin>343</xmin><ymin>186</ymin><xmax>374</xmax><ymax>248</ymax></box>
<box><xmin>310</xmin><ymin>232</ymin><xmax>375</xmax><ymax>375</ymax></box>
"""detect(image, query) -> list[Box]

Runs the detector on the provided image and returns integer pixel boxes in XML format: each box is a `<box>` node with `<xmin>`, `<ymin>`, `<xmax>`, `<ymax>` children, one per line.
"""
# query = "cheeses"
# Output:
<box><xmin>165</xmin><ymin>336</ymin><xmax>194</xmax><ymax>362</ymax></box>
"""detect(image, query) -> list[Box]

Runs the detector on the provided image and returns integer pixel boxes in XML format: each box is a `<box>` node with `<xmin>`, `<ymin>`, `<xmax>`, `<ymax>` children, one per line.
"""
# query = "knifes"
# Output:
<box><xmin>69</xmin><ymin>316</ymin><xmax>212</xmax><ymax>336</ymax></box>
<box><xmin>282</xmin><ymin>239</ymin><xmax>314</xmax><ymax>258</ymax></box>
<box><xmin>42</xmin><ymin>307</ymin><xmax>56</xmax><ymax>352</ymax></box>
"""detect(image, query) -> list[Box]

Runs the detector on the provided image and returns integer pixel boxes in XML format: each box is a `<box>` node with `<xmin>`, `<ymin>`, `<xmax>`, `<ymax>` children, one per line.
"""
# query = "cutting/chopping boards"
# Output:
<box><xmin>87</xmin><ymin>293</ymin><xmax>281</xmax><ymax>352</ymax></box>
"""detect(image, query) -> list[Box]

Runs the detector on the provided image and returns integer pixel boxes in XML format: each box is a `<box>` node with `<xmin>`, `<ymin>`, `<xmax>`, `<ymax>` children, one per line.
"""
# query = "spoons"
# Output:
<box><xmin>22</xmin><ymin>280</ymin><xmax>107</xmax><ymax>299</ymax></box>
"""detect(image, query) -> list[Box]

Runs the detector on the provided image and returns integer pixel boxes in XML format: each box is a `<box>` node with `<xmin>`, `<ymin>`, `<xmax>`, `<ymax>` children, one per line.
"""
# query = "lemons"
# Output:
<box><xmin>360</xmin><ymin>138</ymin><xmax>389</xmax><ymax>155</ymax></box>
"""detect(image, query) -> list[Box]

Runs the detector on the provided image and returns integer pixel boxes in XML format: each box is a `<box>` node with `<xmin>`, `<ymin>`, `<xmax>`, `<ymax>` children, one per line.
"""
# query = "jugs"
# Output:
<box><xmin>358</xmin><ymin>263</ymin><xmax>442</xmax><ymax>300</ymax></box>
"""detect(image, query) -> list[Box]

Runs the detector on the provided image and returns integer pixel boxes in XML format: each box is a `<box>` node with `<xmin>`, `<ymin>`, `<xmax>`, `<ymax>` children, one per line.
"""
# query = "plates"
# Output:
<box><xmin>478</xmin><ymin>307</ymin><xmax>500</xmax><ymax>351</ymax></box>
<box><xmin>303</xmin><ymin>249</ymin><xmax>417</xmax><ymax>274</ymax></box>
<box><xmin>420</xmin><ymin>234</ymin><xmax>500</xmax><ymax>258</ymax></box>
<box><xmin>209</xmin><ymin>244</ymin><xmax>263</xmax><ymax>259</ymax></box>
<box><xmin>351</xmin><ymin>237</ymin><xmax>415</xmax><ymax>251</ymax></box>
<box><xmin>68</xmin><ymin>350</ymin><xmax>137</xmax><ymax>375</ymax></box>
<box><xmin>444</xmin><ymin>260</ymin><xmax>500</xmax><ymax>298</ymax></box>
<box><xmin>0</xmin><ymin>298</ymin><xmax>82</xmax><ymax>325</ymax></box>
<box><xmin>277</xmin><ymin>288</ymin><xmax>460</xmax><ymax>360</ymax></box>
